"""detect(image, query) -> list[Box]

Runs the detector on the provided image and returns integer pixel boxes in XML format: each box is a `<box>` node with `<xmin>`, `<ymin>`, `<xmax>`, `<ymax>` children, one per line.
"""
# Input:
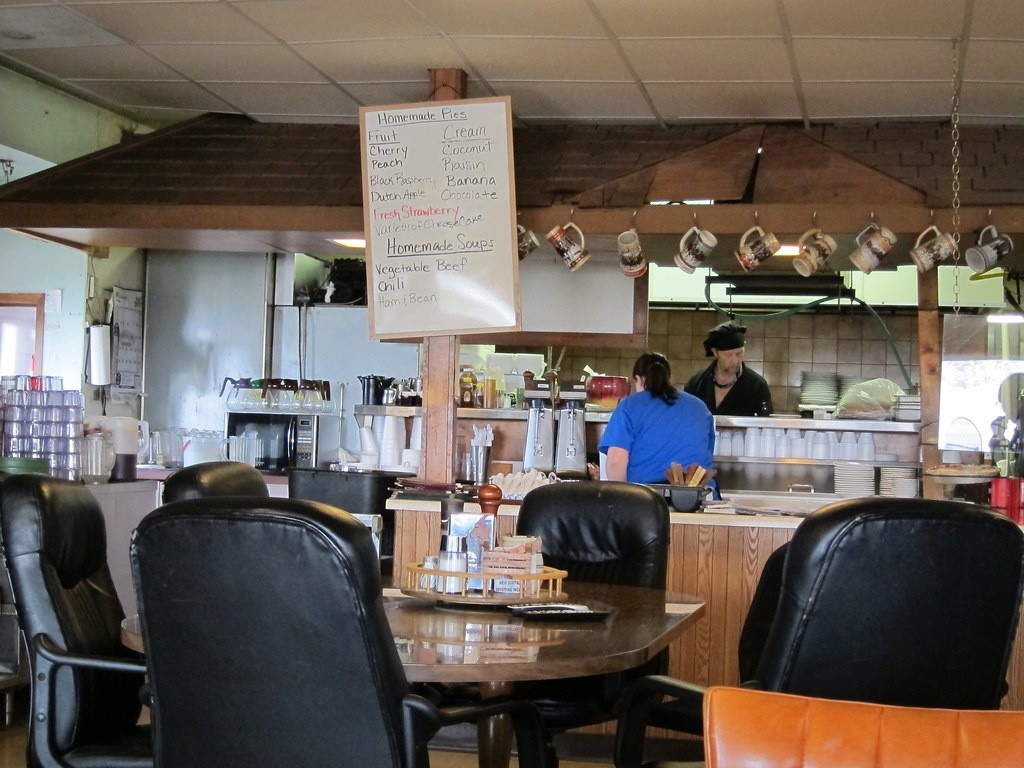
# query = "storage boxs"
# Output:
<box><xmin>285</xmin><ymin>467</ymin><xmax>417</xmax><ymax>557</ymax></box>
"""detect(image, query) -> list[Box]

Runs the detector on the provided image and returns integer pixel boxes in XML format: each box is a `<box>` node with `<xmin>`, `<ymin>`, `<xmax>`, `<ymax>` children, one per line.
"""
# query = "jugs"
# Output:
<box><xmin>356</xmin><ymin>373</ymin><xmax>395</xmax><ymax>405</ymax></box>
<box><xmin>218</xmin><ymin>375</ymin><xmax>336</xmax><ymax>413</ymax></box>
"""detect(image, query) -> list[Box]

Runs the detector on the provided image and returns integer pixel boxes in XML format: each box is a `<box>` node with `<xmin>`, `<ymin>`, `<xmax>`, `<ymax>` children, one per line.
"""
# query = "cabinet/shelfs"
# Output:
<box><xmin>51</xmin><ymin>474</ymin><xmax>158</xmax><ymax>616</ymax></box>
<box><xmin>116</xmin><ymin>579</ymin><xmax>707</xmax><ymax>768</ymax></box>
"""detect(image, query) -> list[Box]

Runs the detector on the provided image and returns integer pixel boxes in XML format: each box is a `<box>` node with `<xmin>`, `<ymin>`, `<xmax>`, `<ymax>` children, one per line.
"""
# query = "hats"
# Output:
<box><xmin>703</xmin><ymin>320</ymin><xmax>747</xmax><ymax>357</ymax></box>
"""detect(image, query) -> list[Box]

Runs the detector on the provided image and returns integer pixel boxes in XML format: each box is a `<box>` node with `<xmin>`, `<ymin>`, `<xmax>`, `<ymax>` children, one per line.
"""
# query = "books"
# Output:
<box><xmin>700</xmin><ymin>501</ymin><xmax>736</xmax><ymax>514</ymax></box>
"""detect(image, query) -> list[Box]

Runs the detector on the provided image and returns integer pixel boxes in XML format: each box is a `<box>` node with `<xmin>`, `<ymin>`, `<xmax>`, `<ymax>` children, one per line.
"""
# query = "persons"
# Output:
<box><xmin>683</xmin><ymin>320</ymin><xmax>774</xmax><ymax>417</ymax></box>
<box><xmin>597</xmin><ymin>352</ymin><xmax>722</xmax><ymax>501</ymax></box>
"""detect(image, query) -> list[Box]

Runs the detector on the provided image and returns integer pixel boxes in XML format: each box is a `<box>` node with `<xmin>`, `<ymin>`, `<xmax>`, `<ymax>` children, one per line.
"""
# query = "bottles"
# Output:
<box><xmin>460</xmin><ymin>368</ymin><xmax>483</xmax><ymax>408</ymax></box>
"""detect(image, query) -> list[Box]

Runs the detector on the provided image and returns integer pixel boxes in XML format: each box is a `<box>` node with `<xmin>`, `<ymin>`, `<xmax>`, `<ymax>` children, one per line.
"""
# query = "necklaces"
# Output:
<box><xmin>712</xmin><ymin>372</ymin><xmax>740</xmax><ymax>390</ymax></box>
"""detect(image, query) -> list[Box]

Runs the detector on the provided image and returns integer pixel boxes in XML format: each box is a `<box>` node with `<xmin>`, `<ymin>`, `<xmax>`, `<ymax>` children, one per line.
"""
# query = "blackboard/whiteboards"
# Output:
<box><xmin>359</xmin><ymin>95</ymin><xmax>521</xmax><ymax>339</ymax></box>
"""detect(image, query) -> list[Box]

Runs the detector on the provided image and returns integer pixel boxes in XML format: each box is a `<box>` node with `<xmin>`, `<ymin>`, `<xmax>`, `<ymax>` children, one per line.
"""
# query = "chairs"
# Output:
<box><xmin>0</xmin><ymin>458</ymin><xmax>1022</xmax><ymax>768</ymax></box>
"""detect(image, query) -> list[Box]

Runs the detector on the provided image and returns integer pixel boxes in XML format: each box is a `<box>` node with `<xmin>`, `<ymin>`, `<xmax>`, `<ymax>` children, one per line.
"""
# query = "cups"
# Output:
<box><xmin>454</xmin><ymin>436</ymin><xmax>473</xmax><ymax>481</ymax></box>
<box><xmin>381</xmin><ymin>415</ymin><xmax>400</xmax><ymax>466</ymax></box>
<box><xmin>470</xmin><ymin>445</ymin><xmax>490</xmax><ymax>483</ymax></box>
<box><xmin>358</xmin><ymin>426</ymin><xmax>380</xmax><ymax>464</ymax></box>
<box><xmin>0</xmin><ymin>374</ymin><xmax>85</xmax><ymax>470</ymax></box>
<box><xmin>137</xmin><ymin>426</ymin><xmax>224</xmax><ymax>467</ymax></box>
<box><xmin>733</xmin><ymin>225</ymin><xmax>782</xmax><ymax>273</ymax></box>
<box><xmin>847</xmin><ymin>222</ymin><xmax>899</xmax><ymax>276</ymax></box>
<box><xmin>483</xmin><ymin>379</ymin><xmax>497</xmax><ymax>409</ymax></box>
<box><xmin>673</xmin><ymin>225</ymin><xmax>718</xmax><ymax>275</ymax></box>
<box><xmin>106</xmin><ymin>416</ymin><xmax>150</xmax><ymax>480</ymax></box>
<box><xmin>79</xmin><ymin>429</ymin><xmax>117</xmax><ymax>479</ymax></box>
<box><xmin>329</xmin><ymin>463</ymin><xmax>372</xmax><ymax>473</ymax></box>
<box><xmin>908</xmin><ymin>225</ymin><xmax>959</xmax><ymax>273</ymax></box>
<box><xmin>391</xmin><ymin>375</ymin><xmax>423</xmax><ymax>407</ymax></box>
<box><xmin>712</xmin><ymin>427</ymin><xmax>898</xmax><ymax>462</ymax></box>
<box><xmin>399</xmin><ymin>416</ymin><xmax>423</xmax><ymax>466</ymax></box>
<box><xmin>670</xmin><ymin>485</ymin><xmax>704</xmax><ymax>511</ymax></box>
<box><xmin>382</xmin><ymin>386</ymin><xmax>397</xmax><ymax>406</ymax></box>
<box><xmin>516</xmin><ymin>225</ymin><xmax>541</xmax><ymax>262</ymax></box>
<box><xmin>964</xmin><ymin>225</ymin><xmax>1014</xmax><ymax>273</ymax></box>
<box><xmin>617</xmin><ymin>227</ymin><xmax>648</xmax><ymax>278</ymax></box>
<box><xmin>791</xmin><ymin>228</ymin><xmax>839</xmax><ymax>278</ymax></box>
<box><xmin>545</xmin><ymin>220</ymin><xmax>591</xmax><ymax>273</ymax></box>
<box><xmin>218</xmin><ymin>435</ymin><xmax>256</xmax><ymax>468</ymax></box>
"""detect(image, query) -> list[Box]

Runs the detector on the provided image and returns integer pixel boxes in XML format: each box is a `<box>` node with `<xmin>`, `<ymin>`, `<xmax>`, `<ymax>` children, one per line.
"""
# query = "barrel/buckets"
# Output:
<box><xmin>182</xmin><ymin>437</ymin><xmax>221</xmax><ymax>469</ymax></box>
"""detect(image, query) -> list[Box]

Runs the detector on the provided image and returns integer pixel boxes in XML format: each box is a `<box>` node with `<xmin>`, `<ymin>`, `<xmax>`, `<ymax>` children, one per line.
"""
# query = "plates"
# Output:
<box><xmin>833</xmin><ymin>464</ymin><xmax>876</xmax><ymax>494</ymax></box>
<box><xmin>768</xmin><ymin>413</ymin><xmax>802</xmax><ymax>418</ymax></box>
<box><xmin>879</xmin><ymin>468</ymin><xmax>918</xmax><ymax>499</ymax></box>
<box><xmin>798</xmin><ymin>370</ymin><xmax>839</xmax><ymax>404</ymax></box>
<box><xmin>508</xmin><ymin>599</ymin><xmax>617</xmax><ymax>621</ymax></box>
<box><xmin>839</xmin><ymin>374</ymin><xmax>877</xmax><ymax>400</ymax></box>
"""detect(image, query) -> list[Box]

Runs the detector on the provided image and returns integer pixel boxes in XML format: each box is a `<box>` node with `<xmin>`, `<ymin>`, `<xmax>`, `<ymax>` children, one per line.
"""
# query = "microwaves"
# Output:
<box><xmin>224</xmin><ymin>411</ymin><xmax>319</xmax><ymax>469</ymax></box>
